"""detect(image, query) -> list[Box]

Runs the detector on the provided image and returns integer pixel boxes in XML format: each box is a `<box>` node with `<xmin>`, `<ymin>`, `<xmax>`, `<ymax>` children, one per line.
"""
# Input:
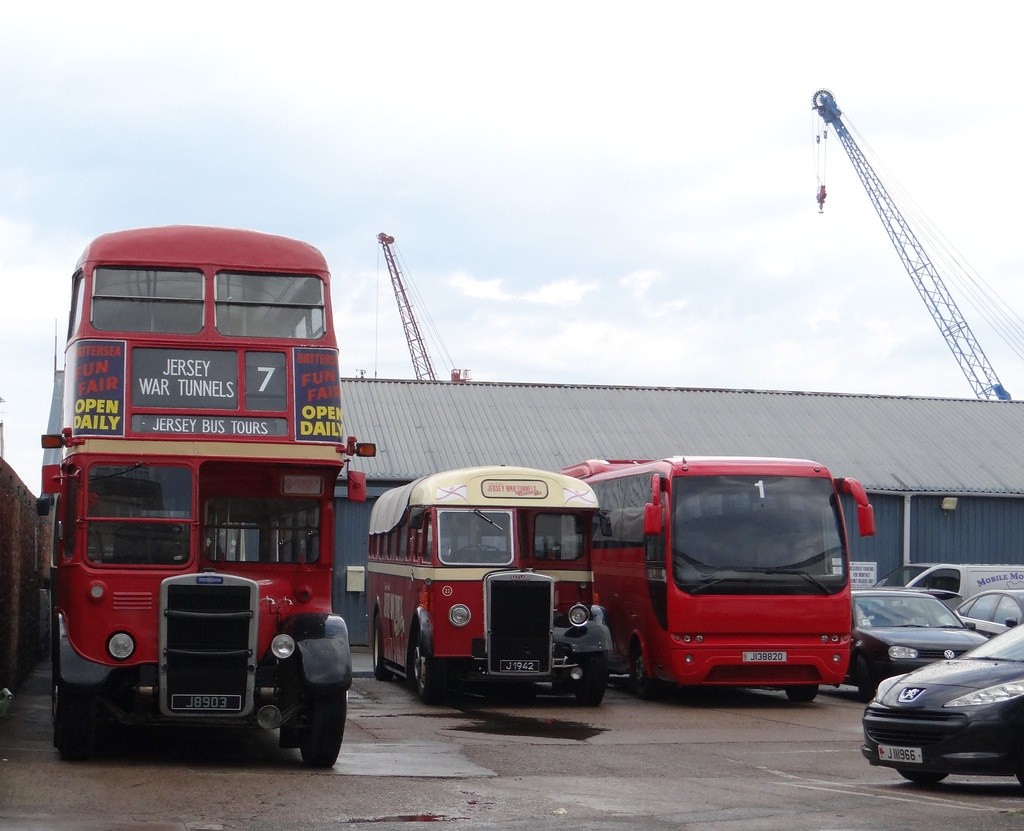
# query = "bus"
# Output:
<box><xmin>574</xmin><ymin>456</ymin><xmax>877</xmax><ymax>698</ymax></box>
<box><xmin>364</xmin><ymin>465</ymin><xmax>614</xmax><ymax>705</ymax></box>
<box><xmin>40</xmin><ymin>225</ymin><xmax>378</xmax><ymax>768</ymax></box>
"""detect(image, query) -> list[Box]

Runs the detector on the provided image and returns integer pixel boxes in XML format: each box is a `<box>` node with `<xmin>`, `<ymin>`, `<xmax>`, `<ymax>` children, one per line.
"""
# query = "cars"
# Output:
<box><xmin>847</xmin><ymin>589</ymin><xmax>989</xmax><ymax>705</ymax></box>
<box><xmin>939</xmin><ymin>592</ymin><xmax>1024</xmax><ymax>639</ymax></box>
<box><xmin>861</xmin><ymin>622</ymin><xmax>1024</xmax><ymax>788</ymax></box>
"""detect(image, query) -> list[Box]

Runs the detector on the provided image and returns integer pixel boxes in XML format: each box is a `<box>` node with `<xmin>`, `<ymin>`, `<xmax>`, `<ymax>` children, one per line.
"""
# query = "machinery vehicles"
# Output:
<box><xmin>380</xmin><ymin>232</ymin><xmax>463</xmax><ymax>382</ymax></box>
<box><xmin>810</xmin><ymin>90</ymin><xmax>1023</xmax><ymax>400</ymax></box>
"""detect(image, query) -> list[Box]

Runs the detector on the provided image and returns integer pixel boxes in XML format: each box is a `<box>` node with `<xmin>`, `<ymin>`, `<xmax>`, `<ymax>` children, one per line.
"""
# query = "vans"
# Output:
<box><xmin>877</xmin><ymin>563</ymin><xmax>1024</xmax><ymax>612</ymax></box>
